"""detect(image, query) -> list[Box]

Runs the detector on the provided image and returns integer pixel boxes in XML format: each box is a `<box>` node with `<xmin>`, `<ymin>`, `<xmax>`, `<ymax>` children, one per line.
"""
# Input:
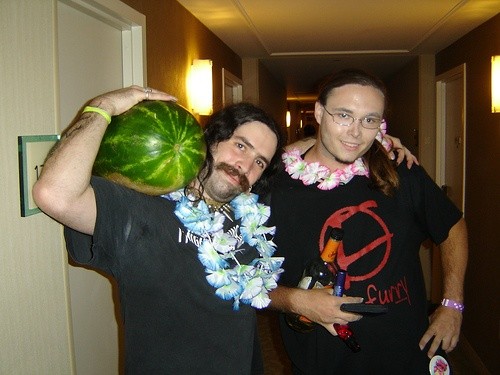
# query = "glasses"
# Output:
<box><xmin>321</xmin><ymin>102</ymin><xmax>384</xmax><ymax>129</ymax></box>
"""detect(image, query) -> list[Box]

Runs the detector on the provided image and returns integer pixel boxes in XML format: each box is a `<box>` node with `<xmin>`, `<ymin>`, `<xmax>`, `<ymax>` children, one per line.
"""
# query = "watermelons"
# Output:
<box><xmin>92</xmin><ymin>100</ymin><xmax>207</xmax><ymax>195</ymax></box>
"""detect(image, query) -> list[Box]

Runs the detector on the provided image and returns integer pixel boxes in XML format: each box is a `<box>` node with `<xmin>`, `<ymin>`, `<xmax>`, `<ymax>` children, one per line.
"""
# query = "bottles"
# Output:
<box><xmin>286</xmin><ymin>226</ymin><xmax>343</xmax><ymax>333</ymax></box>
<box><xmin>334</xmin><ymin>323</ymin><xmax>361</xmax><ymax>354</ymax></box>
<box><xmin>332</xmin><ymin>269</ymin><xmax>345</xmax><ymax>297</ymax></box>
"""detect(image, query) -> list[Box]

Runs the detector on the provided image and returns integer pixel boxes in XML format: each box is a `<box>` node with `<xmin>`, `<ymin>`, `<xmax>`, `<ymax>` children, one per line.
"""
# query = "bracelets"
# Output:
<box><xmin>441</xmin><ymin>298</ymin><xmax>464</xmax><ymax>312</ymax></box>
<box><xmin>81</xmin><ymin>106</ymin><xmax>112</xmax><ymax>124</ymax></box>
<box><xmin>188</xmin><ymin>189</ymin><xmax>223</xmax><ymax>212</ymax></box>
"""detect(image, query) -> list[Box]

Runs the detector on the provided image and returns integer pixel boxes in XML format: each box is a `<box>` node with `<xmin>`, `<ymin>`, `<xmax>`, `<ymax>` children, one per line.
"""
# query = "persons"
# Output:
<box><xmin>252</xmin><ymin>67</ymin><xmax>469</xmax><ymax>375</ymax></box>
<box><xmin>32</xmin><ymin>85</ymin><xmax>421</xmax><ymax>375</ymax></box>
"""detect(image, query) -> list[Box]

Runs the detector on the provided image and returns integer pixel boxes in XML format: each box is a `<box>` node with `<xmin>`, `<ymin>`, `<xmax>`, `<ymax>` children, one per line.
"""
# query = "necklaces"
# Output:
<box><xmin>163</xmin><ymin>183</ymin><xmax>283</xmax><ymax>307</ymax></box>
<box><xmin>284</xmin><ymin>118</ymin><xmax>392</xmax><ymax>188</ymax></box>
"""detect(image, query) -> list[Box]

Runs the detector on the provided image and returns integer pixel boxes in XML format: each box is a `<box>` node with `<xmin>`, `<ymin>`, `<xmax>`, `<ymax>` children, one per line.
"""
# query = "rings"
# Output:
<box><xmin>146</xmin><ymin>93</ymin><xmax>149</xmax><ymax>100</ymax></box>
<box><xmin>145</xmin><ymin>88</ymin><xmax>149</xmax><ymax>92</ymax></box>
